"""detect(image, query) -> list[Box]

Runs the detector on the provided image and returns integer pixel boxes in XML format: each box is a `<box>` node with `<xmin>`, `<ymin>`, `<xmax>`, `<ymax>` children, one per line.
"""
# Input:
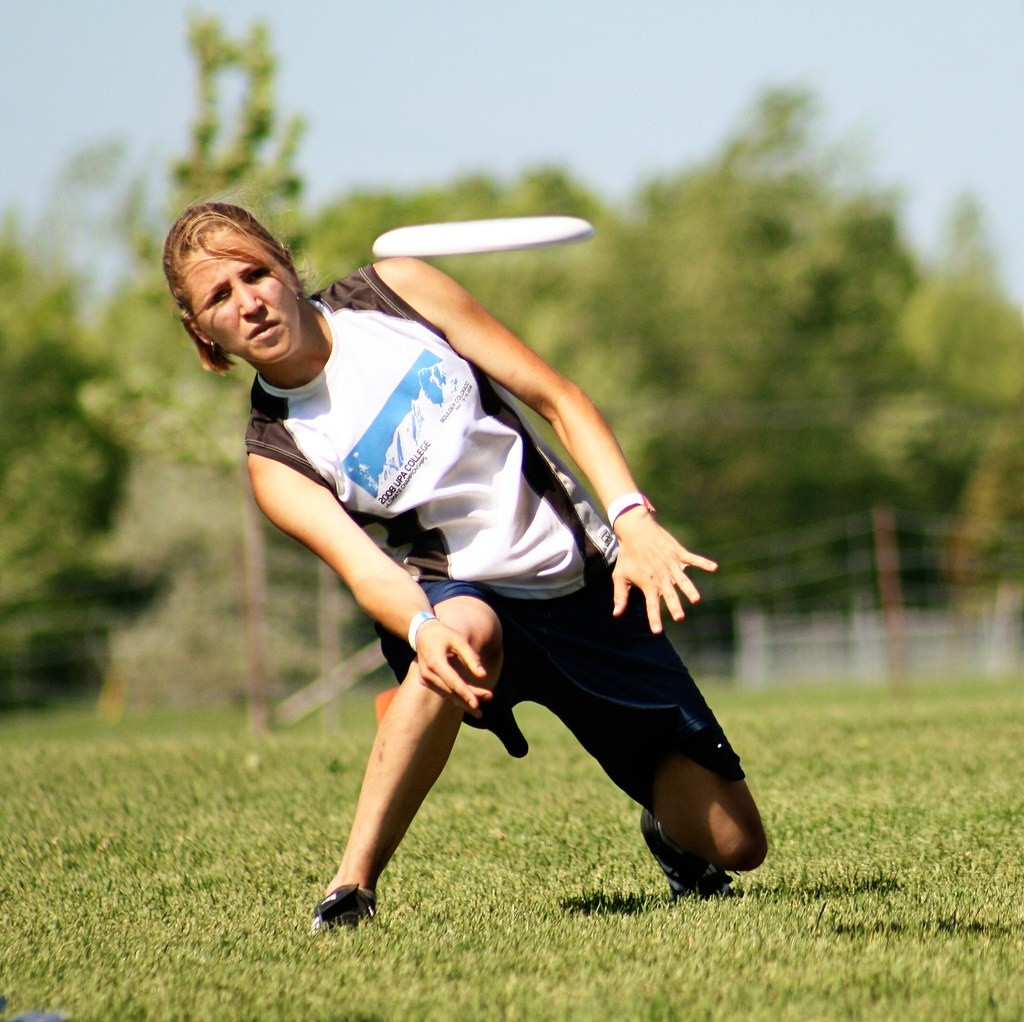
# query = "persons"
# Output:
<box><xmin>162</xmin><ymin>202</ymin><xmax>770</xmax><ymax>931</ymax></box>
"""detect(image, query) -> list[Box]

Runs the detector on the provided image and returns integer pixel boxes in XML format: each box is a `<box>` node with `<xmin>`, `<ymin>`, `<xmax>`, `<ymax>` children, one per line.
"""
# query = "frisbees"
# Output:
<box><xmin>368</xmin><ymin>213</ymin><xmax>597</xmax><ymax>260</ymax></box>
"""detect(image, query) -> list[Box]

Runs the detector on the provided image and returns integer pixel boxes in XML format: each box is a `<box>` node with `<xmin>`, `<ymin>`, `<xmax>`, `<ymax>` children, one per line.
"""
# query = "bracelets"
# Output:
<box><xmin>405</xmin><ymin>610</ymin><xmax>435</xmax><ymax>655</ymax></box>
<box><xmin>606</xmin><ymin>491</ymin><xmax>659</xmax><ymax>533</ymax></box>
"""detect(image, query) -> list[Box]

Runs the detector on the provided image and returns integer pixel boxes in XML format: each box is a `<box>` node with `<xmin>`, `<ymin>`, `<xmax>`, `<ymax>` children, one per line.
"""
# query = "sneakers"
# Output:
<box><xmin>313</xmin><ymin>883</ymin><xmax>377</xmax><ymax>935</ymax></box>
<box><xmin>640</xmin><ymin>807</ymin><xmax>738</xmax><ymax>901</ymax></box>
